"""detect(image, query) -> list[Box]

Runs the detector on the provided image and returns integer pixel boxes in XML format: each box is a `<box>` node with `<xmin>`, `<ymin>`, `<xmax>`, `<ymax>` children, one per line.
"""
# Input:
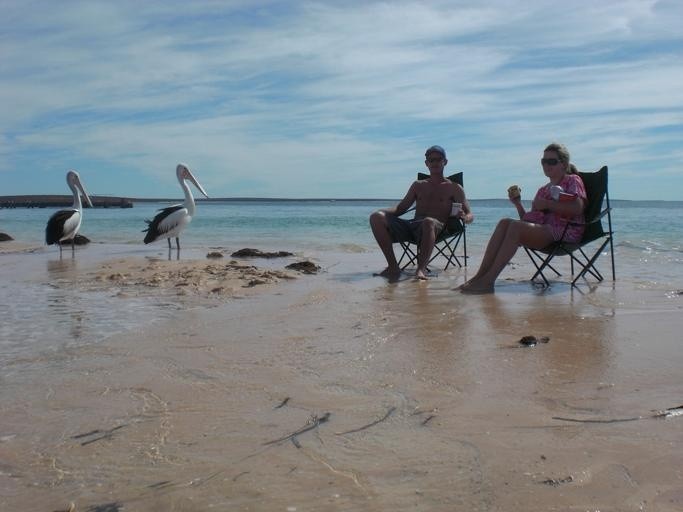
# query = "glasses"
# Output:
<box><xmin>541</xmin><ymin>158</ymin><xmax>557</xmax><ymax>165</ymax></box>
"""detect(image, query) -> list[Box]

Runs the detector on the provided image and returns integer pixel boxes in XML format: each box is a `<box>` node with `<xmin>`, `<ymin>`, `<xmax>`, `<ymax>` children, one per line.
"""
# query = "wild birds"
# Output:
<box><xmin>141</xmin><ymin>164</ymin><xmax>209</xmax><ymax>250</ymax></box>
<box><xmin>46</xmin><ymin>170</ymin><xmax>95</xmax><ymax>251</ymax></box>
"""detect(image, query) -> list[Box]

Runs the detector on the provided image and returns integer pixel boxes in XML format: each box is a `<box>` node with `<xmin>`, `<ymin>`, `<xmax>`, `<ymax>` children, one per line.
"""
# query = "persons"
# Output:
<box><xmin>370</xmin><ymin>145</ymin><xmax>474</xmax><ymax>280</ymax></box>
<box><xmin>453</xmin><ymin>143</ymin><xmax>588</xmax><ymax>292</ymax></box>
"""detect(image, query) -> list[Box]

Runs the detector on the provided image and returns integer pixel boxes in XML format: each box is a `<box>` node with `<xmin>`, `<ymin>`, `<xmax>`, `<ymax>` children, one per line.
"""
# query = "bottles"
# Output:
<box><xmin>547</xmin><ymin>183</ymin><xmax>563</xmax><ymax>201</ymax></box>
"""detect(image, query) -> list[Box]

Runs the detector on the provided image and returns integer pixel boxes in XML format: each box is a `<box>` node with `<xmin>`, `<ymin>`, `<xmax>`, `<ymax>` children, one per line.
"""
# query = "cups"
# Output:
<box><xmin>451</xmin><ymin>202</ymin><xmax>461</xmax><ymax>215</ymax></box>
<box><xmin>508</xmin><ymin>185</ymin><xmax>522</xmax><ymax>201</ymax></box>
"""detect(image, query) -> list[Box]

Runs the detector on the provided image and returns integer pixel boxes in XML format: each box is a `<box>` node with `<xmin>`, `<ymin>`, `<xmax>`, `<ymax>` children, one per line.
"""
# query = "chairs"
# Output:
<box><xmin>393</xmin><ymin>171</ymin><xmax>466</xmax><ymax>272</ymax></box>
<box><xmin>523</xmin><ymin>165</ymin><xmax>615</xmax><ymax>286</ymax></box>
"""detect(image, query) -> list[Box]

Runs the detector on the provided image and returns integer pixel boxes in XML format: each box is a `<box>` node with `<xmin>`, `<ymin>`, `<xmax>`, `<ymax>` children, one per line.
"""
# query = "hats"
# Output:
<box><xmin>425</xmin><ymin>145</ymin><xmax>445</xmax><ymax>157</ymax></box>
